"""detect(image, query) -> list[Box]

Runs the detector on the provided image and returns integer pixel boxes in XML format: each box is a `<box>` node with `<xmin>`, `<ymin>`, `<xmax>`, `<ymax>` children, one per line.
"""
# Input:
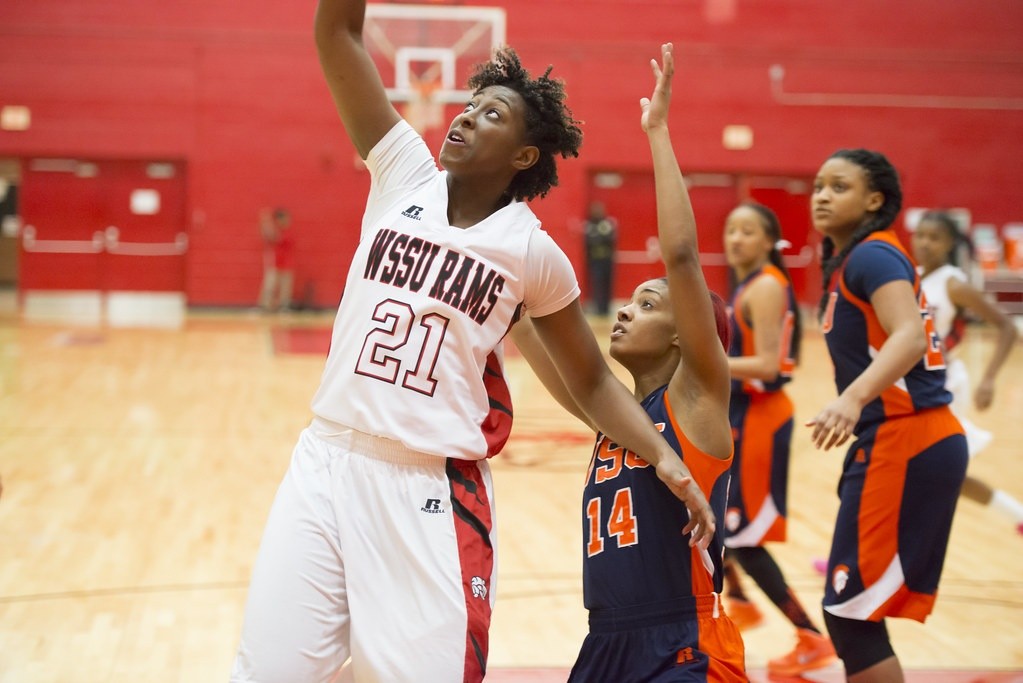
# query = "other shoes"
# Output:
<box><xmin>813</xmin><ymin>560</ymin><xmax>829</xmax><ymax>575</ymax></box>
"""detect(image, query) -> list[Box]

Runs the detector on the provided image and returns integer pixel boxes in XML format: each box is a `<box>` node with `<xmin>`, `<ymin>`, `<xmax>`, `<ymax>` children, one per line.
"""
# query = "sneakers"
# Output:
<box><xmin>767</xmin><ymin>628</ymin><xmax>839</xmax><ymax>676</ymax></box>
<box><xmin>725</xmin><ymin>598</ymin><xmax>763</xmax><ymax>632</ymax></box>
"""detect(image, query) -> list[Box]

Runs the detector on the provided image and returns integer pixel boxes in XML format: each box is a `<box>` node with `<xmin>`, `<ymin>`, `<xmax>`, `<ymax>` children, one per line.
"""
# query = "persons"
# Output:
<box><xmin>457</xmin><ymin>43</ymin><xmax>1023</xmax><ymax>682</ymax></box>
<box><xmin>223</xmin><ymin>0</ymin><xmax>718</xmax><ymax>683</ymax></box>
